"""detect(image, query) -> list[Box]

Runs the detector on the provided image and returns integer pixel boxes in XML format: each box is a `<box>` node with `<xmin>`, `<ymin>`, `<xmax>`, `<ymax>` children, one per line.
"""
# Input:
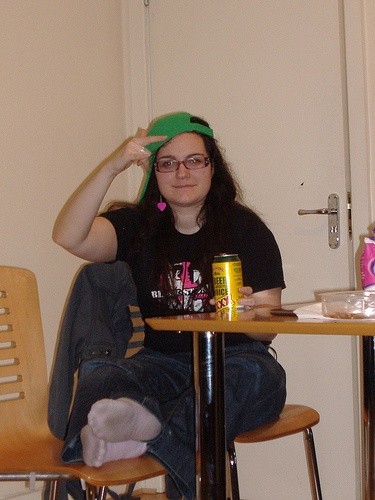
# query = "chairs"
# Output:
<box><xmin>101</xmin><ymin>198</ymin><xmax>324</xmax><ymax>500</ymax></box>
<box><xmin>0</xmin><ymin>264</ymin><xmax>167</xmax><ymax>500</ymax></box>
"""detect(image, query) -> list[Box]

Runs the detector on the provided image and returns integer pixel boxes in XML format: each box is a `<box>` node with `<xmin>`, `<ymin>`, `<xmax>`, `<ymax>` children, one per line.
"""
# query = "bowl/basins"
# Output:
<box><xmin>318</xmin><ymin>291</ymin><xmax>375</xmax><ymax>319</ymax></box>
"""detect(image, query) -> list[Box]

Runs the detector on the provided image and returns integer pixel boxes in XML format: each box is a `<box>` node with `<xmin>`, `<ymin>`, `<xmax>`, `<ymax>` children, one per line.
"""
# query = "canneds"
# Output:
<box><xmin>212</xmin><ymin>253</ymin><xmax>244</xmax><ymax>321</ymax></box>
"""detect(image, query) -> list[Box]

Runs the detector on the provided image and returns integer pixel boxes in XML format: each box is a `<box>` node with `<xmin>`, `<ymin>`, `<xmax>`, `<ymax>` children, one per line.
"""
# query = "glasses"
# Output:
<box><xmin>154</xmin><ymin>156</ymin><xmax>212</xmax><ymax>173</ymax></box>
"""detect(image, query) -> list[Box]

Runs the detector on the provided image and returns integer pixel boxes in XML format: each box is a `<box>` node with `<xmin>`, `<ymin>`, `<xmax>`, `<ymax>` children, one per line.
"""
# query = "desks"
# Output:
<box><xmin>143</xmin><ymin>299</ymin><xmax>375</xmax><ymax>500</ymax></box>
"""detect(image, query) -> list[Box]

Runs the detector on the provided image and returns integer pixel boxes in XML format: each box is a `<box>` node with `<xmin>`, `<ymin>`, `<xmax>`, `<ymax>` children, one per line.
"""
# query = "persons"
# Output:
<box><xmin>56</xmin><ymin>111</ymin><xmax>287</xmax><ymax>467</ymax></box>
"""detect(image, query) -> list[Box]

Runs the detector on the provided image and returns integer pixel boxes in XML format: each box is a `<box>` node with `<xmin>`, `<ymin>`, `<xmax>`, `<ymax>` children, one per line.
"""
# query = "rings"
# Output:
<box><xmin>140</xmin><ymin>146</ymin><xmax>145</xmax><ymax>152</ymax></box>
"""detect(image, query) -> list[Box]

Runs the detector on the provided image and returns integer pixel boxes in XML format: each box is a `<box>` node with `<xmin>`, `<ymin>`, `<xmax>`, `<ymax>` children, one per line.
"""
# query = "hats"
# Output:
<box><xmin>137</xmin><ymin>112</ymin><xmax>215</xmax><ymax>198</ymax></box>
